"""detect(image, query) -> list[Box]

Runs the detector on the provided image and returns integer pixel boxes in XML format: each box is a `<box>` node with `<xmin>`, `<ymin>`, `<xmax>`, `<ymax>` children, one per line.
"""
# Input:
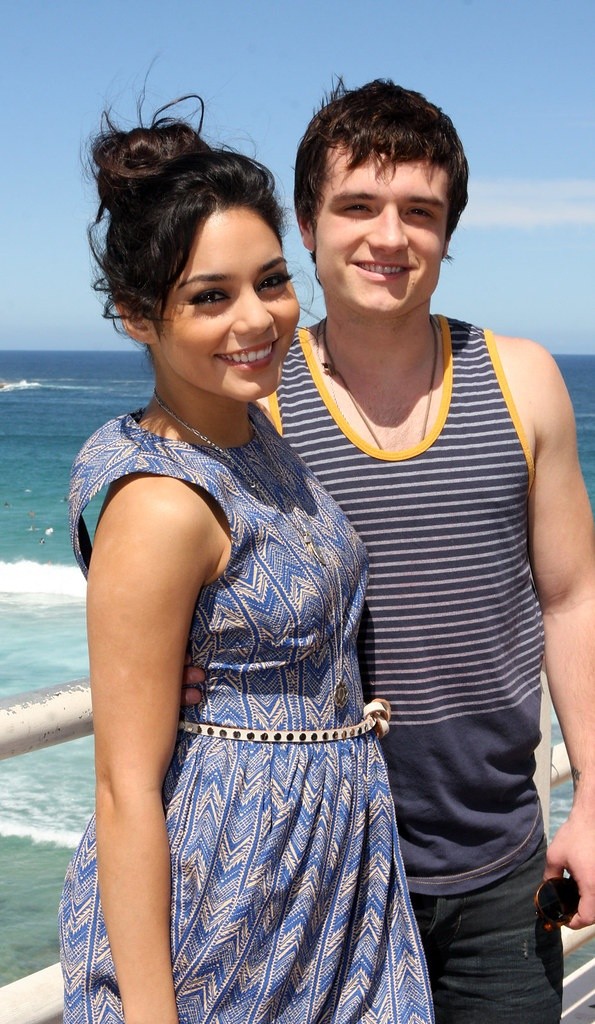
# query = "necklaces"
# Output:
<box><xmin>153</xmin><ymin>388</ymin><xmax>327</xmax><ymax>566</ymax></box>
<box><xmin>321</xmin><ymin>314</ymin><xmax>439</xmax><ymax>450</ymax></box>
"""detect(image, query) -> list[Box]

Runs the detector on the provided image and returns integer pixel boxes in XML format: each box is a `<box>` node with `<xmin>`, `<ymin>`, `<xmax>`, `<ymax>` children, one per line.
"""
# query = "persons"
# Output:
<box><xmin>180</xmin><ymin>77</ymin><xmax>595</xmax><ymax>1024</ymax></box>
<box><xmin>57</xmin><ymin>91</ymin><xmax>433</xmax><ymax>1024</ymax></box>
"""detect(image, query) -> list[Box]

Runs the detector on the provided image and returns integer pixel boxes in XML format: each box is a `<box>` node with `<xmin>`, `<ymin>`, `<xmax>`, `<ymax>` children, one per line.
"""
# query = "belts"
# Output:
<box><xmin>181</xmin><ymin>696</ymin><xmax>392</xmax><ymax>743</ymax></box>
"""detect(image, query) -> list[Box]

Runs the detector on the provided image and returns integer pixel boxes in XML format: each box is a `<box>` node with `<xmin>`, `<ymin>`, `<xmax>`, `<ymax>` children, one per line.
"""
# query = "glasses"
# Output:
<box><xmin>534</xmin><ymin>875</ymin><xmax>581</xmax><ymax>929</ymax></box>
